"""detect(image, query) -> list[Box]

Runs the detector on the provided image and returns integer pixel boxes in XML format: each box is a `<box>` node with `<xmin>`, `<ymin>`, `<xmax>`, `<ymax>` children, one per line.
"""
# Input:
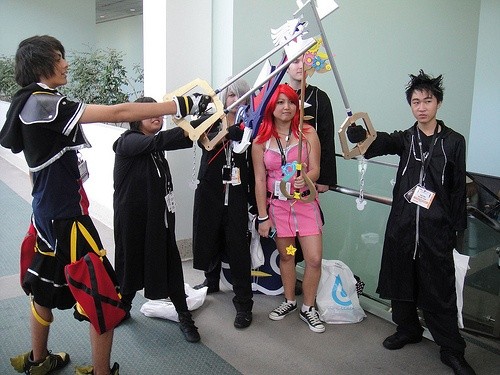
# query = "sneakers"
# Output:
<box><xmin>269</xmin><ymin>298</ymin><xmax>298</xmax><ymax>320</ymax></box>
<box><xmin>299</xmin><ymin>304</ymin><xmax>325</xmax><ymax>333</ymax></box>
<box><xmin>75</xmin><ymin>361</ymin><xmax>120</xmax><ymax>375</ymax></box>
<box><xmin>9</xmin><ymin>349</ymin><xmax>71</xmax><ymax>375</ymax></box>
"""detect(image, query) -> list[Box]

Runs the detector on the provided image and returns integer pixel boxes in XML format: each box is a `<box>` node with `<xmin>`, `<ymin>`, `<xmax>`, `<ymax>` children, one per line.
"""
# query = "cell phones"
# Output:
<box><xmin>222</xmin><ymin>165</ymin><xmax>232</xmax><ymax>184</ymax></box>
<box><xmin>269</xmin><ymin>227</ymin><xmax>277</xmax><ymax>239</ymax></box>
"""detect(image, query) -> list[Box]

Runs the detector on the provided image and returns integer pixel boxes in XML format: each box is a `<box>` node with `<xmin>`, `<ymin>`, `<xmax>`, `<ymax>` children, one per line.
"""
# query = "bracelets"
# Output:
<box><xmin>258</xmin><ymin>215</ymin><xmax>268</xmax><ymax>220</ymax></box>
<box><xmin>257</xmin><ymin>217</ymin><xmax>269</xmax><ymax>223</ymax></box>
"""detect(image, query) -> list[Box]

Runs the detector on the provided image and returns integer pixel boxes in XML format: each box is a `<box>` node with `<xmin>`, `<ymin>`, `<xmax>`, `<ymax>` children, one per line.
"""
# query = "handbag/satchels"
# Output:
<box><xmin>316</xmin><ymin>258</ymin><xmax>367</xmax><ymax>324</ymax></box>
<box><xmin>60</xmin><ymin>218</ymin><xmax>127</xmax><ymax>335</ymax></box>
<box><xmin>139</xmin><ymin>281</ymin><xmax>208</xmax><ymax>322</ymax></box>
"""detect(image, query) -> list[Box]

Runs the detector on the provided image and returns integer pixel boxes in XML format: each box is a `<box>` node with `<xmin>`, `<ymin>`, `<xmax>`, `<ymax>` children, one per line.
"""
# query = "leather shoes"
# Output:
<box><xmin>440</xmin><ymin>349</ymin><xmax>477</xmax><ymax>375</ymax></box>
<box><xmin>383</xmin><ymin>329</ymin><xmax>423</xmax><ymax>350</ymax></box>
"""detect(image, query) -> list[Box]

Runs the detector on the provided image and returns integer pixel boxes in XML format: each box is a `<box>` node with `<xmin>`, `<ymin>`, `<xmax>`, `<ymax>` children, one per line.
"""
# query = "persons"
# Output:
<box><xmin>192</xmin><ymin>76</ymin><xmax>254</xmax><ymax>328</ymax></box>
<box><xmin>279</xmin><ymin>56</ymin><xmax>337</xmax><ymax>265</ymax></box>
<box><xmin>347</xmin><ymin>69</ymin><xmax>476</xmax><ymax>375</ymax></box>
<box><xmin>113</xmin><ymin>98</ymin><xmax>222</xmax><ymax>344</ymax></box>
<box><xmin>256</xmin><ymin>87</ymin><xmax>325</xmax><ymax>333</ymax></box>
<box><xmin>0</xmin><ymin>35</ymin><xmax>213</xmax><ymax>375</ymax></box>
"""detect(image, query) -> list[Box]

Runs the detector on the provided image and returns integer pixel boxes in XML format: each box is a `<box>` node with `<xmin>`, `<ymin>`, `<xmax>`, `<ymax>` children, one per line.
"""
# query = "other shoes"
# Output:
<box><xmin>234</xmin><ymin>310</ymin><xmax>252</xmax><ymax>328</ymax></box>
<box><xmin>192</xmin><ymin>278</ymin><xmax>219</xmax><ymax>294</ymax></box>
<box><xmin>113</xmin><ymin>301</ymin><xmax>133</xmax><ymax>328</ymax></box>
<box><xmin>179</xmin><ymin>319</ymin><xmax>201</xmax><ymax>343</ymax></box>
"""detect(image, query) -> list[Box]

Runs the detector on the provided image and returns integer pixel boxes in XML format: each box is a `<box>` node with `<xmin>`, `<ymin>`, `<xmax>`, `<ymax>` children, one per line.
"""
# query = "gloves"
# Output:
<box><xmin>225</xmin><ymin>122</ymin><xmax>244</xmax><ymax>141</ymax></box>
<box><xmin>347</xmin><ymin>124</ymin><xmax>367</xmax><ymax>143</ymax></box>
<box><xmin>173</xmin><ymin>92</ymin><xmax>212</xmax><ymax>120</ymax></box>
<box><xmin>191</xmin><ymin>114</ymin><xmax>223</xmax><ymax>133</ymax></box>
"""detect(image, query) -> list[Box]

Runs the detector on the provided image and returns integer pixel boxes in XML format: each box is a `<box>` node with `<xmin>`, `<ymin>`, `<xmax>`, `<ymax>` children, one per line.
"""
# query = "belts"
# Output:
<box><xmin>267</xmin><ymin>185</ymin><xmax>318</xmax><ymax>199</ymax></box>
